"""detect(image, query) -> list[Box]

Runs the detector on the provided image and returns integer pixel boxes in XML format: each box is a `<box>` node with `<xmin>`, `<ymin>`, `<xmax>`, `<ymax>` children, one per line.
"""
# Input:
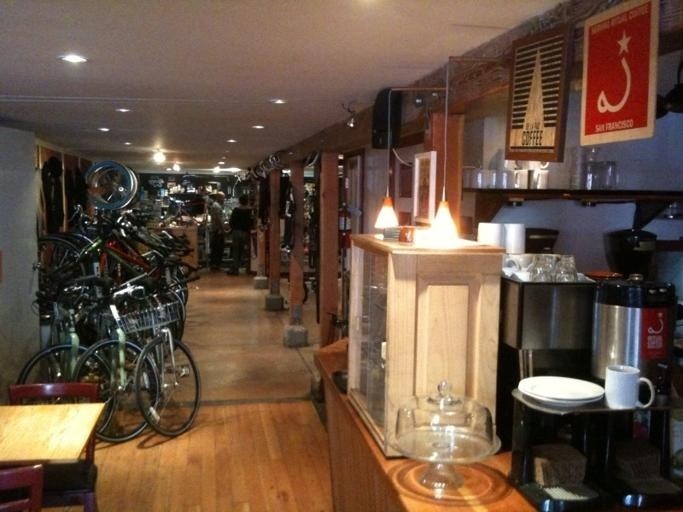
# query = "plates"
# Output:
<box><xmin>518</xmin><ymin>375</ymin><xmax>604</xmax><ymax>407</ymax></box>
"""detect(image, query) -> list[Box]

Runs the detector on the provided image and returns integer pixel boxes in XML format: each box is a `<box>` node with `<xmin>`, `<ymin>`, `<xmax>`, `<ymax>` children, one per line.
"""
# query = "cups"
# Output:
<box><xmin>463</xmin><ymin>160</ymin><xmax>550</xmax><ymax>189</ymax></box>
<box><xmin>585</xmin><ymin>161</ymin><xmax>616</xmax><ymax>188</ymax></box>
<box><xmin>504</xmin><ymin>252</ymin><xmax>577</xmax><ymax>282</ymax></box>
<box><xmin>604</xmin><ymin>365</ymin><xmax>655</xmax><ymax>411</ymax></box>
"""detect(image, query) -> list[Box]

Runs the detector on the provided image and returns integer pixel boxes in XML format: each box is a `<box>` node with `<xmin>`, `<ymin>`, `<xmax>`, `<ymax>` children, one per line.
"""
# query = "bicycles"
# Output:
<box><xmin>160</xmin><ymin>200</ymin><xmax>234</xmax><ymax>233</ymax></box>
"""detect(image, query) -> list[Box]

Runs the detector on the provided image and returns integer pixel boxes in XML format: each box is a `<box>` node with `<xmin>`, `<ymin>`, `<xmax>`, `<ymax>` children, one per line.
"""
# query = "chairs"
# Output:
<box><xmin>0</xmin><ymin>381</ymin><xmax>100</xmax><ymax>512</ymax></box>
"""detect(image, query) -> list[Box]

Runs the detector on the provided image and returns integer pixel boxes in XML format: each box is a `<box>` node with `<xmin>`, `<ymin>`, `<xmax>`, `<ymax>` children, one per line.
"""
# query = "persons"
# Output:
<box><xmin>227</xmin><ymin>194</ymin><xmax>257</xmax><ymax>277</ymax></box>
<box><xmin>209</xmin><ymin>191</ymin><xmax>227</xmax><ymax>272</ymax></box>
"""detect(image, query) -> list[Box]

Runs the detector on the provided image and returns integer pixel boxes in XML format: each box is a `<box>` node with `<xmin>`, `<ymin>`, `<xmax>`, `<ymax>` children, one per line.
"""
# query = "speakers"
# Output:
<box><xmin>372</xmin><ymin>88</ymin><xmax>401</xmax><ymax>149</ymax></box>
